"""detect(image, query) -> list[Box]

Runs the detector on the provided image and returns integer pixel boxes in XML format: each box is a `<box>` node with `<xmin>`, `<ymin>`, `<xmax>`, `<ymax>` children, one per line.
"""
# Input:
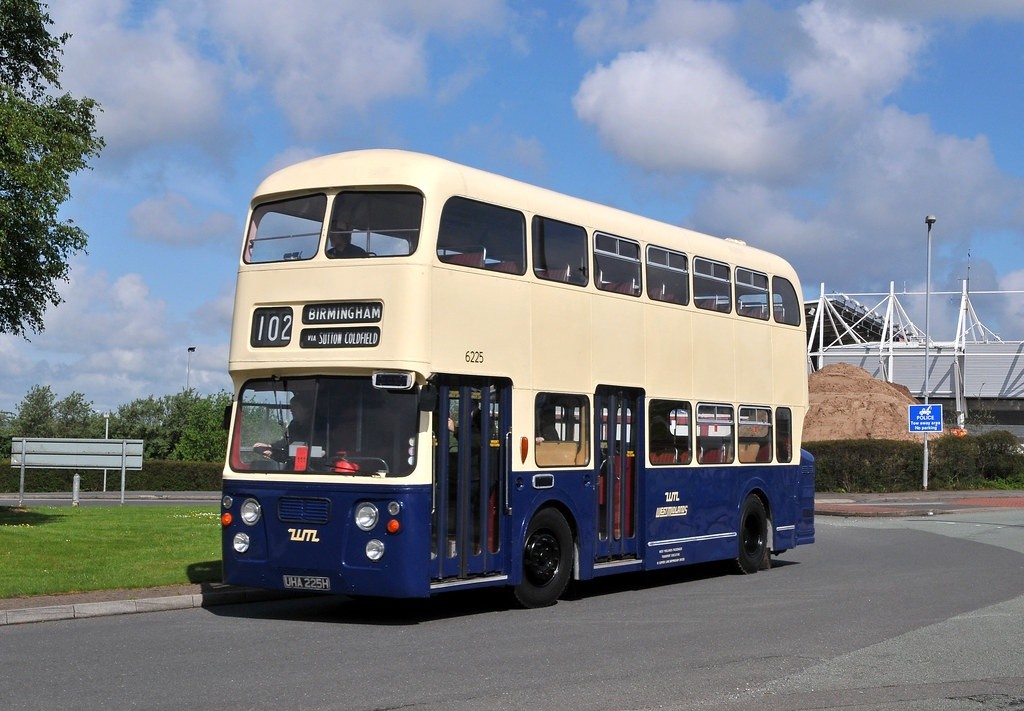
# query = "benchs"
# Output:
<box><xmin>437</xmin><ymin>246</ymin><xmax>785</xmax><ymax>323</ymax></box>
<box><xmin>650</xmin><ymin>442</ymin><xmax>791</xmax><ymax>464</ymax></box>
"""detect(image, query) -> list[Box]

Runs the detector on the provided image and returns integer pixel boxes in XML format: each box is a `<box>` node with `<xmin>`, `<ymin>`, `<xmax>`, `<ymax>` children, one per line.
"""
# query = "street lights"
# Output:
<box><xmin>921</xmin><ymin>214</ymin><xmax>936</xmax><ymax>494</ymax></box>
<box><xmin>186</xmin><ymin>346</ymin><xmax>195</xmax><ymax>390</ymax></box>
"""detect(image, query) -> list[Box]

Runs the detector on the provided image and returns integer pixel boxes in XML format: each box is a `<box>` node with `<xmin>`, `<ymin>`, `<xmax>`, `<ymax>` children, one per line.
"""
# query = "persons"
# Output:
<box><xmin>447</xmin><ymin>398</ymin><xmax>498</xmax><ymax>528</ymax></box>
<box><xmin>324</xmin><ymin>217</ymin><xmax>368</xmax><ymax>259</ymax></box>
<box><xmin>253</xmin><ymin>395</ymin><xmax>325</xmax><ymax>462</ymax></box>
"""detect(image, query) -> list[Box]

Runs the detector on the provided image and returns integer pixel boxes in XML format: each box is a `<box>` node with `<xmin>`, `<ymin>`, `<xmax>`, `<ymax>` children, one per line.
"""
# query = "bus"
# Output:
<box><xmin>221</xmin><ymin>149</ymin><xmax>815</xmax><ymax>607</ymax></box>
